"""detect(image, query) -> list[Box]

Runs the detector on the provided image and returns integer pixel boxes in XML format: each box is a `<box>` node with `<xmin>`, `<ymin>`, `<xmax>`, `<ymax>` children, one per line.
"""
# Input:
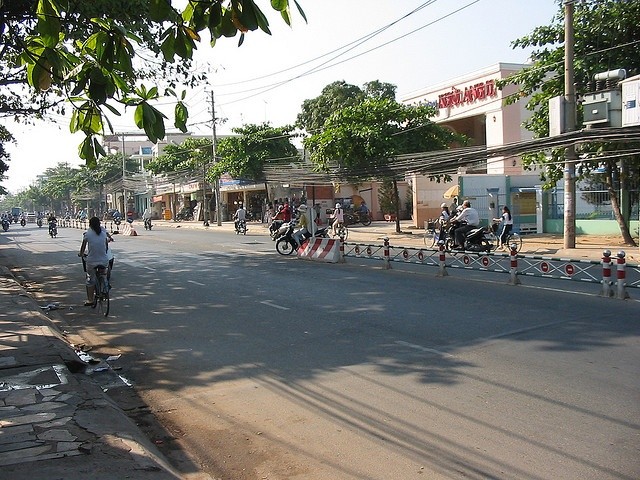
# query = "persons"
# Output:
<box><xmin>313</xmin><ymin>203</ymin><xmax>321</xmax><ymax>225</ymax></box>
<box><xmin>439</xmin><ymin>198</ymin><xmax>479</xmax><ymax>243</ymax></box>
<box><xmin>76</xmin><ymin>210</ymin><xmax>87</xmax><ymax>222</ymax></box>
<box><xmin>113</xmin><ymin>209</ymin><xmax>121</xmax><ymax>219</ymax></box>
<box><xmin>19</xmin><ymin>212</ymin><xmax>25</xmax><ymax>219</ymax></box>
<box><xmin>493</xmin><ymin>206</ymin><xmax>513</xmax><ymax>249</ymax></box>
<box><xmin>80</xmin><ymin>217</ymin><xmax>112</xmax><ymax>303</ymax></box>
<box><xmin>142</xmin><ymin>209</ymin><xmax>151</xmax><ymax>227</ymax></box>
<box><xmin>357</xmin><ymin>201</ymin><xmax>369</xmax><ymax>214</ymax></box>
<box><xmin>233</xmin><ymin>205</ymin><xmax>245</xmax><ymax>231</ymax></box>
<box><xmin>0</xmin><ymin>213</ymin><xmax>18</xmax><ymax>227</ymax></box>
<box><xmin>37</xmin><ymin>212</ymin><xmax>43</xmax><ymax>220</ymax></box>
<box><xmin>281</xmin><ymin>204</ymin><xmax>290</xmax><ymax>222</ymax></box>
<box><xmin>330</xmin><ymin>203</ymin><xmax>343</xmax><ymax>236</ymax></box>
<box><xmin>291</xmin><ymin>205</ymin><xmax>308</xmax><ymax>250</ymax></box>
<box><xmin>48</xmin><ymin>214</ymin><xmax>57</xmax><ymax>234</ymax></box>
<box><xmin>271</xmin><ymin>205</ymin><xmax>283</xmax><ymax>220</ymax></box>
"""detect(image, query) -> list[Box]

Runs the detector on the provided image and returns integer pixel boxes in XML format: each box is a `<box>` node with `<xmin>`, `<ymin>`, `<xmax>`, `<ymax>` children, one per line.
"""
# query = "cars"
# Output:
<box><xmin>26</xmin><ymin>212</ymin><xmax>35</xmax><ymax>216</ymax></box>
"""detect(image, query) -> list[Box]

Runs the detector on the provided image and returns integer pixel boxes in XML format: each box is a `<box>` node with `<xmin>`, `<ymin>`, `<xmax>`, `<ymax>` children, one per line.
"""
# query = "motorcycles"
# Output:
<box><xmin>233</xmin><ymin>214</ymin><xmax>249</xmax><ymax>235</ymax></box>
<box><xmin>269</xmin><ymin>218</ymin><xmax>293</xmax><ymax>241</ymax></box>
<box><xmin>49</xmin><ymin>221</ymin><xmax>58</xmax><ymax>238</ymax></box>
<box><xmin>444</xmin><ymin>220</ymin><xmax>494</xmax><ymax>257</ymax></box>
<box><xmin>114</xmin><ymin>216</ymin><xmax>121</xmax><ymax>225</ymax></box>
<box><xmin>37</xmin><ymin>217</ymin><xmax>42</xmax><ymax>227</ymax></box>
<box><xmin>2</xmin><ymin>219</ymin><xmax>9</xmax><ymax>232</ymax></box>
<box><xmin>276</xmin><ymin>220</ymin><xmax>331</xmax><ymax>255</ymax></box>
<box><xmin>13</xmin><ymin>215</ymin><xmax>18</xmax><ymax>222</ymax></box>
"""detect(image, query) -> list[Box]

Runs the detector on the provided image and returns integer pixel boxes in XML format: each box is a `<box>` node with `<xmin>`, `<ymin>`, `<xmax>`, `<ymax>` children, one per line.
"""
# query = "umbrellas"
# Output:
<box><xmin>444</xmin><ymin>185</ymin><xmax>458</xmax><ymax>199</ymax></box>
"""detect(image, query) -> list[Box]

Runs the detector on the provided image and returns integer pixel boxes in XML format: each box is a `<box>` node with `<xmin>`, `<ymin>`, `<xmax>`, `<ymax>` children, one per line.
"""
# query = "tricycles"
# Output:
<box><xmin>342</xmin><ymin>209</ymin><xmax>371</xmax><ymax>227</ymax></box>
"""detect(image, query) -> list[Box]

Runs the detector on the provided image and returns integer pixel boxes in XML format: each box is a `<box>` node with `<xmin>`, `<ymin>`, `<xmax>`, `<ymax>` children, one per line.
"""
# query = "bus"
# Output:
<box><xmin>10</xmin><ymin>207</ymin><xmax>22</xmax><ymax>220</ymax></box>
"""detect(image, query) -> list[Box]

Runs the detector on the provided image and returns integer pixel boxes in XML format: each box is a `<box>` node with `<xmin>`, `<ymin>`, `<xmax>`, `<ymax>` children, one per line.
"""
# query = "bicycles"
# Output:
<box><xmin>8</xmin><ymin>216</ymin><xmax>12</xmax><ymax>224</ymax></box>
<box><xmin>424</xmin><ymin>218</ymin><xmax>452</xmax><ymax>248</ymax></box>
<box><xmin>487</xmin><ymin>218</ymin><xmax>521</xmax><ymax>252</ymax></box>
<box><xmin>20</xmin><ymin>218</ymin><xmax>26</xmax><ymax>227</ymax></box>
<box><xmin>144</xmin><ymin>218</ymin><xmax>153</xmax><ymax>230</ymax></box>
<box><xmin>326</xmin><ymin>217</ymin><xmax>348</xmax><ymax>241</ymax></box>
<box><xmin>78</xmin><ymin>254</ymin><xmax>110</xmax><ymax>317</ymax></box>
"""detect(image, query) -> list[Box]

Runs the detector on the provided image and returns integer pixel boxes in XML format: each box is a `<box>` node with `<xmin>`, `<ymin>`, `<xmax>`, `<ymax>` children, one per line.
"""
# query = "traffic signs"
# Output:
<box><xmin>125</xmin><ymin>204</ymin><xmax>133</xmax><ymax>223</ymax></box>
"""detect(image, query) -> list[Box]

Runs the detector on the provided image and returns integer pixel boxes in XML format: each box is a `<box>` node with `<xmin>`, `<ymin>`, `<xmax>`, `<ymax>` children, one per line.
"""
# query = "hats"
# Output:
<box><xmin>335</xmin><ymin>203</ymin><xmax>341</xmax><ymax>208</ymax></box>
<box><xmin>297</xmin><ymin>204</ymin><xmax>308</xmax><ymax>213</ymax></box>
